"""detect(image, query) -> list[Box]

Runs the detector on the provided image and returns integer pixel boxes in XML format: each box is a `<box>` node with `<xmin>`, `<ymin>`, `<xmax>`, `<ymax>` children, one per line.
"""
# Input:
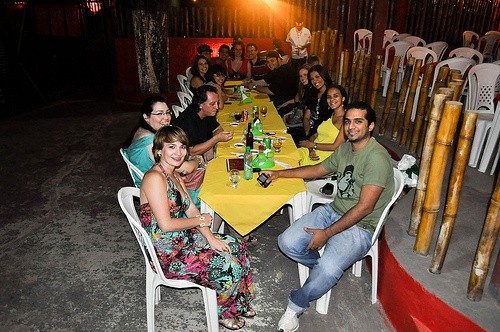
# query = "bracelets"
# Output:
<box><xmin>195</xmin><ymin>158</ymin><xmax>201</xmax><ymax>167</ymax></box>
<box><xmin>199</xmin><ymin>214</ymin><xmax>206</xmax><ymax>229</ymax></box>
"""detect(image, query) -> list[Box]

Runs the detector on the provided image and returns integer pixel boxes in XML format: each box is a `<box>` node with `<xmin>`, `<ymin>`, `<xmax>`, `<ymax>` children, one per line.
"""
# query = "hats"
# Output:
<box><xmin>198</xmin><ymin>44</ymin><xmax>213</xmax><ymax>54</ymax></box>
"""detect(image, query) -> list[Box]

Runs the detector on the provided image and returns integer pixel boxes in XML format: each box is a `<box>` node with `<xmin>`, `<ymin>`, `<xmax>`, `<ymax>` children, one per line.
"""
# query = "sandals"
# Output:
<box><xmin>241</xmin><ymin>308</ymin><xmax>256</xmax><ymax>318</ymax></box>
<box><xmin>218</xmin><ymin>316</ymin><xmax>245</xmax><ymax>330</ymax></box>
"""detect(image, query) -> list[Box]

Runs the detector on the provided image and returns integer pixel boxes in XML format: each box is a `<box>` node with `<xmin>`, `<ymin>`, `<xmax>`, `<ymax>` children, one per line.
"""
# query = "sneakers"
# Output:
<box><xmin>276</xmin><ymin>306</ymin><xmax>300</xmax><ymax>332</ymax></box>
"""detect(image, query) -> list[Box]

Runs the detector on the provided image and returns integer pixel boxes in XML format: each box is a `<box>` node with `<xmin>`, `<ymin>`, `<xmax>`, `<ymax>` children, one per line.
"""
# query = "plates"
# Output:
<box><xmin>250</xmin><ymin>149</ymin><xmax>259</xmax><ymax>155</ymax></box>
<box><xmin>234</xmin><ymin>142</ymin><xmax>244</xmax><ymax>148</ymax></box>
<box><xmin>236</xmin><ymin>153</ymin><xmax>244</xmax><ymax>159</ymax></box>
<box><xmin>231</xmin><ymin>123</ymin><xmax>239</xmax><ymax>126</ymax></box>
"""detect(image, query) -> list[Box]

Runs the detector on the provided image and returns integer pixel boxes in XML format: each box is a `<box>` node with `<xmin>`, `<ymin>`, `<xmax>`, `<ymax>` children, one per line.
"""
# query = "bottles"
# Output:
<box><xmin>243</xmin><ymin>146</ymin><xmax>254</xmax><ymax>180</ymax></box>
<box><xmin>252</xmin><ymin>111</ymin><xmax>261</xmax><ymax>127</ymax></box>
<box><xmin>251</xmin><ymin>145</ymin><xmax>276</xmax><ymax>169</ymax></box>
<box><xmin>246</xmin><ymin>122</ymin><xmax>253</xmax><ymax>151</ymax></box>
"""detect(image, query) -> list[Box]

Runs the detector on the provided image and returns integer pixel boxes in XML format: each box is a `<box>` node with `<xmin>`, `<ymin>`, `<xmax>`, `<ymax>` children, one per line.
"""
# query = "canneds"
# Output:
<box><xmin>252</xmin><ymin>105</ymin><xmax>259</xmax><ymax>118</ymax></box>
<box><xmin>263</xmin><ymin>136</ymin><xmax>271</xmax><ymax>150</ymax></box>
<box><xmin>242</xmin><ymin>110</ymin><xmax>248</xmax><ymax>122</ymax></box>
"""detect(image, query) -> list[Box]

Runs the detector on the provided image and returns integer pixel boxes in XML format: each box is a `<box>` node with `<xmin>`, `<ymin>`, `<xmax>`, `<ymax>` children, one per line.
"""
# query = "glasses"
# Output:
<box><xmin>151</xmin><ymin>111</ymin><xmax>173</xmax><ymax>116</ymax></box>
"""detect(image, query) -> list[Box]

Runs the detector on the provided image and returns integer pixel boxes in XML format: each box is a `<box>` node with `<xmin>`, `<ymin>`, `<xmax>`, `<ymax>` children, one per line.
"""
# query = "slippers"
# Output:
<box><xmin>246</xmin><ymin>235</ymin><xmax>257</xmax><ymax>242</ymax></box>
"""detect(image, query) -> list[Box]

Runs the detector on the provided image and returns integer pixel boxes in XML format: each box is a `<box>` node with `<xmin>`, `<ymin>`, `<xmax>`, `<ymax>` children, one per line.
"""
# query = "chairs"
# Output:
<box><xmin>316</xmin><ymin>167</ymin><xmax>404</xmax><ymax>315</ymax></box>
<box><xmin>307</xmin><ymin>174</ymin><xmax>337</xmax><ymax>212</ymax></box>
<box><xmin>117</xmin><ymin>186</ymin><xmax>219</xmax><ymax>332</ymax></box>
<box><xmin>353</xmin><ymin>29</ymin><xmax>500</xmax><ymax>175</ymax></box>
<box><xmin>120</xmin><ymin>148</ymin><xmax>144</xmax><ymax>188</ymax></box>
<box><xmin>172</xmin><ymin>67</ymin><xmax>194</xmax><ymax>118</ymax></box>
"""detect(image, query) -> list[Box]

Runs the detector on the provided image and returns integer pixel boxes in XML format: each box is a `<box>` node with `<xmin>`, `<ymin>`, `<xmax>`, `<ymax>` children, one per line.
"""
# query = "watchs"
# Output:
<box><xmin>314</xmin><ymin>143</ymin><xmax>319</xmax><ymax>150</ymax></box>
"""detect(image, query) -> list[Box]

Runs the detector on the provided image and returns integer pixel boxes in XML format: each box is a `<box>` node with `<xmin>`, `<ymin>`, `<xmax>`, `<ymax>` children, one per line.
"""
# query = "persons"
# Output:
<box><xmin>286</xmin><ymin>17</ymin><xmax>312</xmax><ymax>71</ymax></box>
<box><xmin>246</xmin><ymin>44</ymin><xmax>270</xmax><ymax>74</ymax></box>
<box><xmin>257</xmin><ymin>102</ymin><xmax>396</xmax><ymax>332</ymax></box>
<box><xmin>173</xmin><ymin>85</ymin><xmax>233</xmax><ymax>161</ymax></box>
<box><xmin>138</xmin><ymin>126</ymin><xmax>255</xmax><ymax>330</ymax></box>
<box><xmin>125</xmin><ymin>96</ymin><xmax>206</xmax><ymax>209</ymax></box>
<box><xmin>232</xmin><ymin>35</ymin><xmax>242</xmax><ymax>42</ymax></box>
<box><xmin>250</xmin><ymin>50</ymin><xmax>347</xmax><ymax>166</ymax></box>
<box><xmin>225</xmin><ymin>42</ymin><xmax>252</xmax><ymax>83</ymax></box>
<box><xmin>187</xmin><ymin>44</ymin><xmax>232</xmax><ymax>109</ymax></box>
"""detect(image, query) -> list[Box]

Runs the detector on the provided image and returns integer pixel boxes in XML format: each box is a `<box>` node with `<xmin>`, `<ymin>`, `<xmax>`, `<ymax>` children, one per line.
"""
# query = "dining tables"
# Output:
<box><xmin>200</xmin><ymin>81</ymin><xmax>309</xmax><ymax>287</ymax></box>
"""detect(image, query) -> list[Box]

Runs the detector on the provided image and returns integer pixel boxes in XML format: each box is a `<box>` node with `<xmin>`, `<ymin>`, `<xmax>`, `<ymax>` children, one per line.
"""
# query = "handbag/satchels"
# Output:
<box><xmin>182</xmin><ymin>167</ymin><xmax>205</xmax><ymax>191</ymax></box>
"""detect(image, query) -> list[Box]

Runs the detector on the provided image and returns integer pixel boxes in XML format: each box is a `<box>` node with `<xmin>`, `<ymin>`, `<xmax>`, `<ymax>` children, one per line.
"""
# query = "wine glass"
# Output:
<box><xmin>228</xmin><ymin>169</ymin><xmax>240</xmax><ymax>189</ymax></box>
<box><xmin>273</xmin><ymin>137</ymin><xmax>282</xmax><ymax>153</ymax></box>
<box><xmin>260</xmin><ymin>105</ymin><xmax>267</xmax><ymax>118</ymax></box>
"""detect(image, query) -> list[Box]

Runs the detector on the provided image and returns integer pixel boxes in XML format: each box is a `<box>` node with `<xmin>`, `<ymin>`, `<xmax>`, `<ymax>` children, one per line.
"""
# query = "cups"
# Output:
<box><xmin>234</xmin><ymin>112</ymin><xmax>241</xmax><ymax>122</ymax></box>
